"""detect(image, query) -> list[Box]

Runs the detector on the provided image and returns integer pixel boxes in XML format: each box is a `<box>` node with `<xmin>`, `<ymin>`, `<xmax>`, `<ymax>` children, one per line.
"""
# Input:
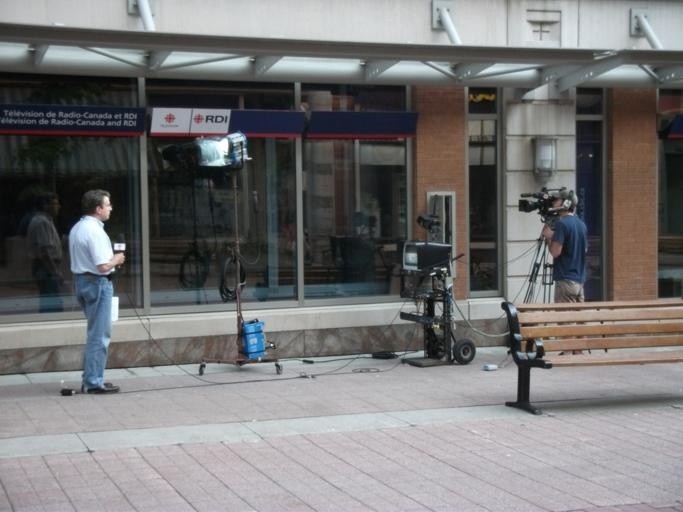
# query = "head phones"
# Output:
<box><xmin>562</xmin><ymin>190</ymin><xmax>574</xmax><ymax>211</ymax></box>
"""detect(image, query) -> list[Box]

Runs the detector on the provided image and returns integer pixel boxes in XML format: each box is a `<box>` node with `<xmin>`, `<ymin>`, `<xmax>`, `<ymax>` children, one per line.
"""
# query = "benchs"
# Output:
<box><xmin>125</xmin><ymin>237</ymin><xmax>215</xmax><ymax>278</ymax></box>
<box><xmin>500</xmin><ymin>298</ymin><xmax>683</xmax><ymax>416</ymax></box>
<box><xmin>254</xmin><ymin>263</ymin><xmax>364</xmax><ymax>302</ymax></box>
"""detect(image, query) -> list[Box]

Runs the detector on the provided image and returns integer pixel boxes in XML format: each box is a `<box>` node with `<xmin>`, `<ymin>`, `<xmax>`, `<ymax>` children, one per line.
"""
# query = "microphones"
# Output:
<box><xmin>113</xmin><ymin>234</ymin><xmax>126</xmax><ymax>271</ymax></box>
<box><xmin>548</xmin><ymin>207</ymin><xmax>564</xmax><ymax>211</ymax></box>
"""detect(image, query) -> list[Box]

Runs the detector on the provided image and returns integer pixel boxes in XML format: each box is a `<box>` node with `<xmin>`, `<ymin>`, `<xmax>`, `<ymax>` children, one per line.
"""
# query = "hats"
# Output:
<box><xmin>551</xmin><ymin>191</ymin><xmax>578</xmax><ymax>206</ymax></box>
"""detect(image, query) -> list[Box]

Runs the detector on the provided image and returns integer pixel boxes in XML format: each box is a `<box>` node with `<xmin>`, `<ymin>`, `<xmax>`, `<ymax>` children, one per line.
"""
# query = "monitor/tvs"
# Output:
<box><xmin>403</xmin><ymin>241</ymin><xmax>453</xmax><ymax>272</ymax></box>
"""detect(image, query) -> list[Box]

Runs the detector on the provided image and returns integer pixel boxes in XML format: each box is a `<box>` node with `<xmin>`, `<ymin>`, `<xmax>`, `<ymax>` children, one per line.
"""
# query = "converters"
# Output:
<box><xmin>303</xmin><ymin>359</ymin><xmax>313</xmax><ymax>364</ymax></box>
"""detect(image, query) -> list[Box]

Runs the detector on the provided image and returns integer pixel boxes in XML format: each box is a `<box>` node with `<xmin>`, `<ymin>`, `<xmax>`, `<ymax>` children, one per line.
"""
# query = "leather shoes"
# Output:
<box><xmin>88</xmin><ymin>384</ymin><xmax>119</xmax><ymax>395</ymax></box>
<box><xmin>82</xmin><ymin>382</ymin><xmax>112</xmax><ymax>393</ymax></box>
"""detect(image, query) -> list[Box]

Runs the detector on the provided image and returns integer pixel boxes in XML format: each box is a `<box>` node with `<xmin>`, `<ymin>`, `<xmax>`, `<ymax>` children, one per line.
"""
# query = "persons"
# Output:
<box><xmin>27</xmin><ymin>193</ymin><xmax>65</xmax><ymax>313</ymax></box>
<box><xmin>542</xmin><ymin>192</ymin><xmax>588</xmax><ymax>356</ymax></box>
<box><xmin>69</xmin><ymin>189</ymin><xmax>125</xmax><ymax>394</ymax></box>
